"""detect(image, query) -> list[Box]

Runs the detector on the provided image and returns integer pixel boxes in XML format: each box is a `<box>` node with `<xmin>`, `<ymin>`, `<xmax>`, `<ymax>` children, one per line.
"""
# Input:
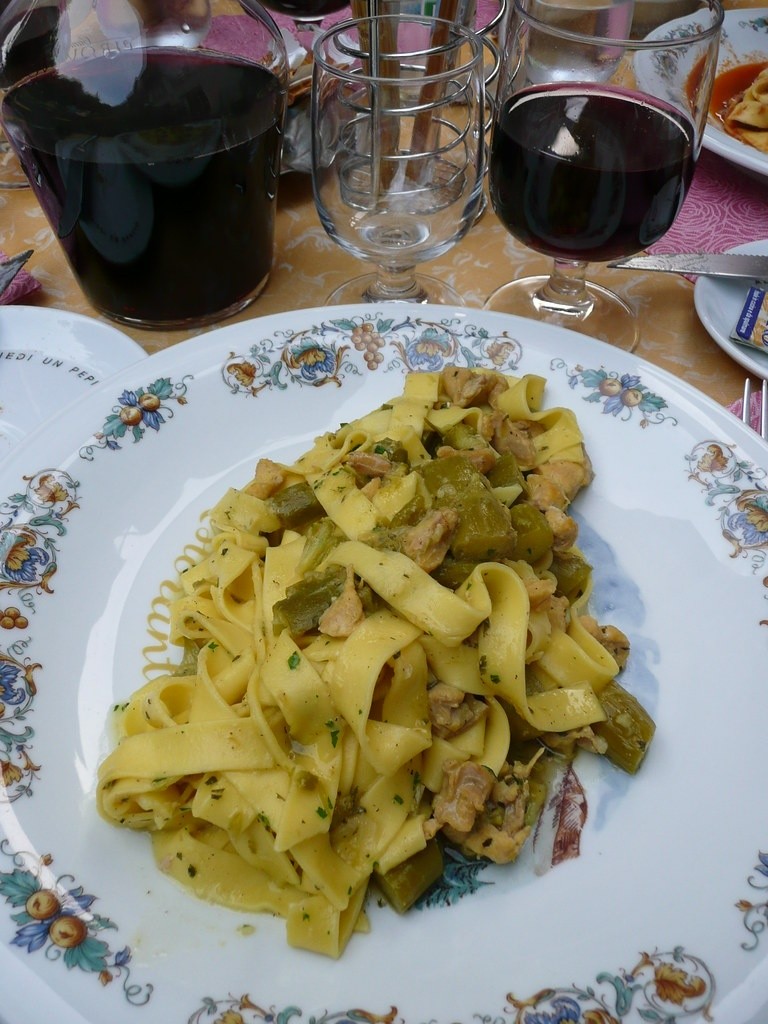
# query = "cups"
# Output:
<box><xmin>519</xmin><ymin>0</ymin><xmax>636</xmax><ymax>85</ymax></box>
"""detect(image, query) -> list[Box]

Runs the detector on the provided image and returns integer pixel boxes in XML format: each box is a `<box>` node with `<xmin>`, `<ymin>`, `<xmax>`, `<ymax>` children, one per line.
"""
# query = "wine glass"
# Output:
<box><xmin>310</xmin><ymin>15</ymin><xmax>484</xmax><ymax>307</ymax></box>
<box><xmin>256</xmin><ymin>0</ymin><xmax>351</xmax><ymax>31</ymax></box>
<box><xmin>481</xmin><ymin>0</ymin><xmax>725</xmax><ymax>353</ymax></box>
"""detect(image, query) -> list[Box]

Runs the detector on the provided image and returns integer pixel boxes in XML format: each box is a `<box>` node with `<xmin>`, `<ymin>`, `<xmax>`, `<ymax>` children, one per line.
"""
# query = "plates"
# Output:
<box><xmin>693</xmin><ymin>238</ymin><xmax>768</xmax><ymax>382</ymax></box>
<box><xmin>0</xmin><ymin>302</ymin><xmax>768</xmax><ymax>1024</ymax></box>
<box><xmin>1</xmin><ymin>305</ymin><xmax>150</xmax><ymax>465</ymax></box>
<box><xmin>632</xmin><ymin>7</ymin><xmax>768</xmax><ymax>176</ymax></box>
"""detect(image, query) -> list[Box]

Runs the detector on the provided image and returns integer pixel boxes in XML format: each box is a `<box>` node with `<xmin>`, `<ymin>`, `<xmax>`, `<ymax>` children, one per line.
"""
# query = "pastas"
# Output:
<box><xmin>93</xmin><ymin>369</ymin><xmax>621</xmax><ymax>959</ymax></box>
<box><xmin>724</xmin><ymin>66</ymin><xmax>768</xmax><ymax>152</ymax></box>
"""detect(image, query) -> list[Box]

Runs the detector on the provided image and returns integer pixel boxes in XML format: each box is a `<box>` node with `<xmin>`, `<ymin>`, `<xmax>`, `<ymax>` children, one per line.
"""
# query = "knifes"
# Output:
<box><xmin>606</xmin><ymin>252</ymin><xmax>768</xmax><ymax>280</ymax></box>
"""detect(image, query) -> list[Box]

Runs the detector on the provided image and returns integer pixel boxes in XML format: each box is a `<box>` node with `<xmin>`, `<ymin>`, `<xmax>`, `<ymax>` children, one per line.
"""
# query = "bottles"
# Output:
<box><xmin>0</xmin><ymin>0</ymin><xmax>288</xmax><ymax>331</ymax></box>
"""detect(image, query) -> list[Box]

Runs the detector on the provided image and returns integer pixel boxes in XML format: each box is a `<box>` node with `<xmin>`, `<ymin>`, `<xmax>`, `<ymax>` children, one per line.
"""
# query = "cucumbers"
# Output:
<box><xmin>268</xmin><ymin>429</ymin><xmax>658</xmax><ymax>912</ymax></box>
